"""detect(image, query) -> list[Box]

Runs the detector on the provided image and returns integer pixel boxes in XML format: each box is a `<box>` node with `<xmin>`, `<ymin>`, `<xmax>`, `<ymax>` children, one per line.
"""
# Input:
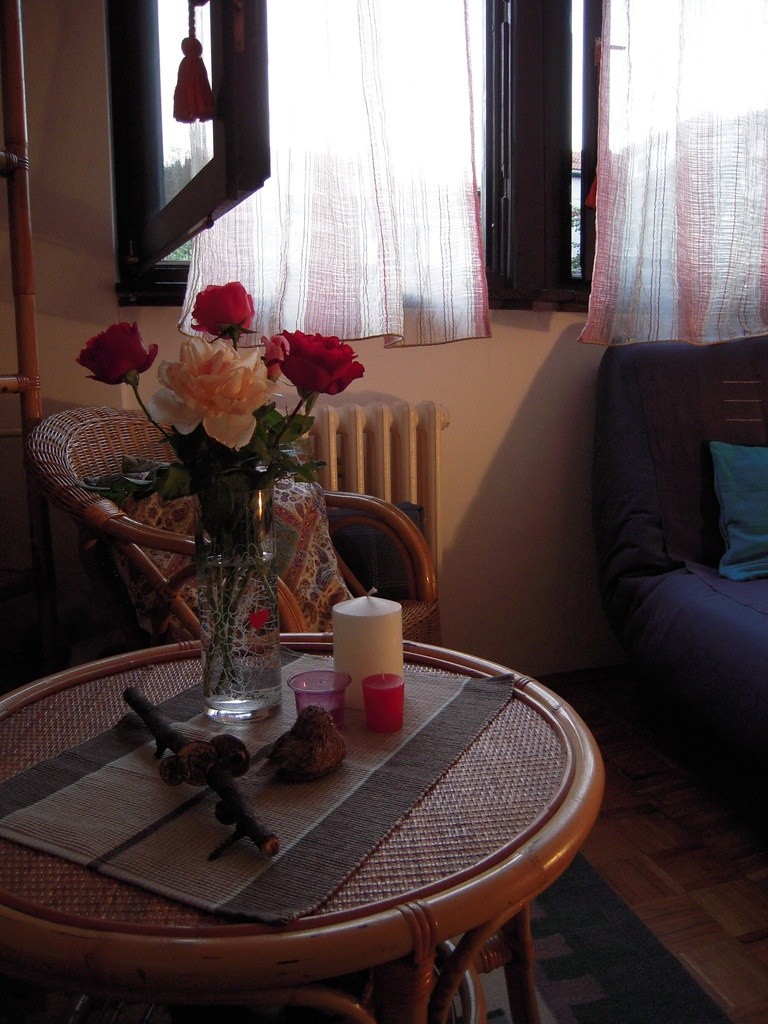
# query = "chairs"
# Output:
<box><xmin>23</xmin><ymin>406</ymin><xmax>445</xmax><ymax>653</ymax></box>
<box><xmin>194</xmin><ymin>464</ymin><xmax>199</xmax><ymax>469</ymax></box>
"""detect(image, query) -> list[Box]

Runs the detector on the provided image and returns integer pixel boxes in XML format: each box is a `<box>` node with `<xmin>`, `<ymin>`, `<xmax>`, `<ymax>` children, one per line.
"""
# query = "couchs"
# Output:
<box><xmin>588</xmin><ymin>333</ymin><xmax>768</xmax><ymax>846</ymax></box>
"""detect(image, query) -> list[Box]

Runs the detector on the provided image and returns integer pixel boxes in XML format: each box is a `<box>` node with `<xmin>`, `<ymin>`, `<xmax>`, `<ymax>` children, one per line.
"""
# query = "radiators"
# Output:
<box><xmin>271</xmin><ymin>401</ymin><xmax>447</xmax><ymax>602</ymax></box>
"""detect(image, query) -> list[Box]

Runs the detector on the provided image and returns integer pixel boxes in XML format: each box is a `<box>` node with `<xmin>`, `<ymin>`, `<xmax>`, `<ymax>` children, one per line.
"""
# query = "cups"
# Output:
<box><xmin>287</xmin><ymin>669</ymin><xmax>352</xmax><ymax>727</ymax></box>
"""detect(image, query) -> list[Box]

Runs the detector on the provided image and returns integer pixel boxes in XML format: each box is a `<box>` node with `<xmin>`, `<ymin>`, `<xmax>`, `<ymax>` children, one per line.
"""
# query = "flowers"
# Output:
<box><xmin>75</xmin><ymin>282</ymin><xmax>364</xmax><ymax>701</ymax></box>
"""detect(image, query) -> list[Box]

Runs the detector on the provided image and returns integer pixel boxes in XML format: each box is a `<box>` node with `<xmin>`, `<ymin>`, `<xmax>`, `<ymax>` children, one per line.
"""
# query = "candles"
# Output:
<box><xmin>362</xmin><ymin>674</ymin><xmax>405</xmax><ymax>733</ymax></box>
<box><xmin>332</xmin><ymin>587</ymin><xmax>403</xmax><ymax>712</ymax></box>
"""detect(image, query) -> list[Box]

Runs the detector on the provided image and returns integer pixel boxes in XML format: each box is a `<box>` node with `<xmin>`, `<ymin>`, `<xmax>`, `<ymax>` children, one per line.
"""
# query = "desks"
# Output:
<box><xmin>0</xmin><ymin>630</ymin><xmax>606</xmax><ymax>1024</ymax></box>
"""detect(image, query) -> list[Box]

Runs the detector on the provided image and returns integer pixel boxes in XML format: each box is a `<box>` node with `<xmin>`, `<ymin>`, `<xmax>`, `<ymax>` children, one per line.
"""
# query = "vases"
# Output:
<box><xmin>196</xmin><ymin>467</ymin><xmax>285</xmax><ymax>724</ymax></box>
